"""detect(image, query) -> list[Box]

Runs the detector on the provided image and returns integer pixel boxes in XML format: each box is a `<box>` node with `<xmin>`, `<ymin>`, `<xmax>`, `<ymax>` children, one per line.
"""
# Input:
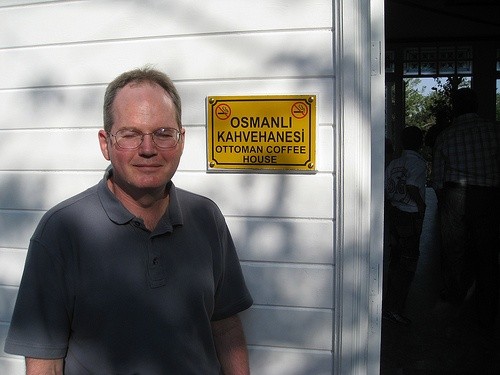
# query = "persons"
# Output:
<box><xmin>3</xmin><ymin>67</ymin><xmax>252</xmax><ymax>375</ymax></box>
<box><xmin>433</xmin><ymin>88</ymin><xmax>500</xmax><ymax>325</ymax></box>
<box><xmin>383</xmin><ymin>126</ymin><xmax>428</xmax><ymax>329</ymax></box>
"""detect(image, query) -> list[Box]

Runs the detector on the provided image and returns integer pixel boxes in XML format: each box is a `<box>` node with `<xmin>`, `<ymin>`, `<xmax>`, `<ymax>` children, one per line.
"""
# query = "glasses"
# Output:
<box><xmin>106</xmin><ymin>125</ymin><xmax>183</xmax><ymax>152</ymax></box>
